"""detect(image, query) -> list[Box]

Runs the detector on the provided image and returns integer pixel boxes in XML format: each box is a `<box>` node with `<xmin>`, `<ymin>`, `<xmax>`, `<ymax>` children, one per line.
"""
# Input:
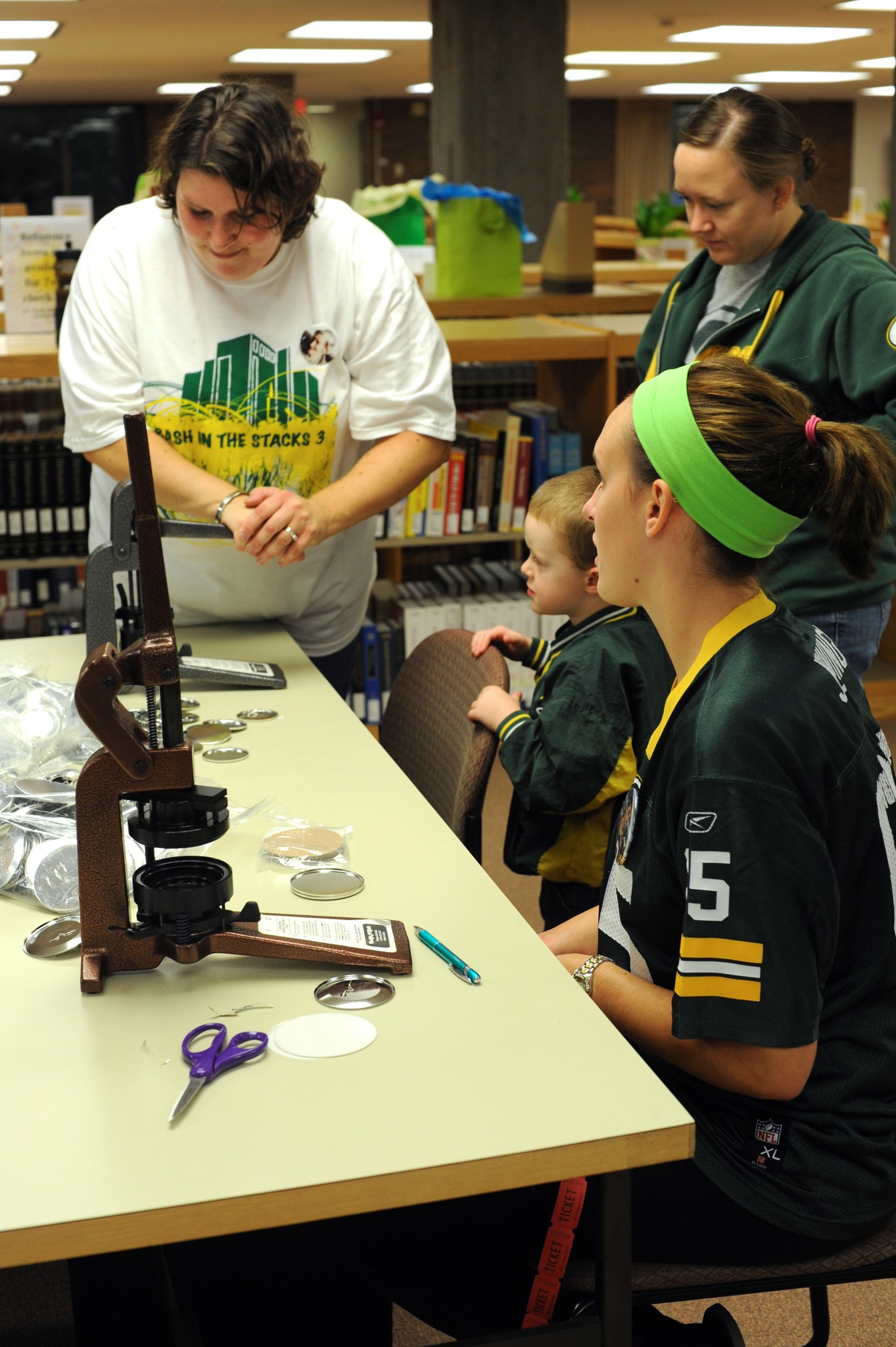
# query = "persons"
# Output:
<box><xmin>394</xmin><ymin>355</ymin><xmax>896</xmax><ymax>1347</ymax></box>
<box><xmin>633</xmin><ymin>86</ymin><xmax>895</xmax><ymax>684</ymax></box>
<box><xmin>468</xmin><ymin>465</ymin><xmax>677</xmax><ymax>931</ymax></box>
<box><xmin>57</xmin><ymin>81</ymin><xmax>458</xmax><ymax>701</ymax></box>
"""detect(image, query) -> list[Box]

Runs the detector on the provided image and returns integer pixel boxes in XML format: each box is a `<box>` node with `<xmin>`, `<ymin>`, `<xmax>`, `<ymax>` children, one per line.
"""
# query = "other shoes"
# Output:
<box><xmin>703</xmin><ymin>1303</ymin><xmax>746</xmax><ymax>1347</ymax></box>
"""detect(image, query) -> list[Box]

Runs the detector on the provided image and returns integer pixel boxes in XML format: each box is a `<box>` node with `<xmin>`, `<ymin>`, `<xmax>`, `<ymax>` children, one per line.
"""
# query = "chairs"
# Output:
<box><xmin>380</xmin><ymin>628</ymin><xmax>505</xmax><ymax>865</ymax></box>
<box><xmin>624</xmin><ymin>1229</ymin><xmax>896</xmax><ymax>1346</ymax></box>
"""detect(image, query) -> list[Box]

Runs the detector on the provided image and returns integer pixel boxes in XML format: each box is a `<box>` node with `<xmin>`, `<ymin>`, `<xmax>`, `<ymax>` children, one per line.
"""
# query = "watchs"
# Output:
<box><xmin>571</xmin><ymin>954</ymin><xmax>615</xmax><ymax>997</ymax></box>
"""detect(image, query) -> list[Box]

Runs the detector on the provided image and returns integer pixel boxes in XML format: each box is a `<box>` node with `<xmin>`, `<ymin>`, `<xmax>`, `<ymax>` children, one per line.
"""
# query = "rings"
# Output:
<box><xmin>283</xmin><ymin>526</ymin><xmax>298</xmax><ymax>541</ymax></box>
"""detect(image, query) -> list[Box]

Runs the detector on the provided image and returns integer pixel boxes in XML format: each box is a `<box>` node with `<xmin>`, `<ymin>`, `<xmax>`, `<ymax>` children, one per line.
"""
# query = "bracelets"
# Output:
<box><xmin>214</xmin><ymin>491</ymin><xmax>249</xmax><ymax>523</ymax></box>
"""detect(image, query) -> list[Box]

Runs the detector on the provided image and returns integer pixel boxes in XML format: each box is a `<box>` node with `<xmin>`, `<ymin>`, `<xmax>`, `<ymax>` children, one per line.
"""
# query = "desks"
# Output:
<box><xmin>0</xmin><ymin>618</ymin><xmax>694</xmax><ymax>1346</ymax></box>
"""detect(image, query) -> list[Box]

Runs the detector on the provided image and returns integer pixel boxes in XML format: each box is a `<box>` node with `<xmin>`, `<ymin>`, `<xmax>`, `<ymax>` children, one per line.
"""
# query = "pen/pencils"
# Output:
<box><xmin>413</xmin><ymin>926</ymin><xmax>481</xmax><ymax>984</ymax></box>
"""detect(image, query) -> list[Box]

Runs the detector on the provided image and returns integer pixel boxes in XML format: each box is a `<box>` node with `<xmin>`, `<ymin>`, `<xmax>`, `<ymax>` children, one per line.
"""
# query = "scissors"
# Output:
<box><xmin>169</xmin><ymin>1023</ymin><xmax>268</xmax><ymax>1122</ymax></box>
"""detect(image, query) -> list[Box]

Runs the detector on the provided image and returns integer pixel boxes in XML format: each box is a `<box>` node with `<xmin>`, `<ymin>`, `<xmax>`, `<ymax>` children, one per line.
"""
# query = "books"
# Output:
<box><xmin>374</xmin><ymin>401</ymin><xmax>581</xmax><ymax>540</ymax></box>
<box><xmin>0</xmin><ymin>426</ymin><xmax>91</xmax><ymax>612</ymax></box>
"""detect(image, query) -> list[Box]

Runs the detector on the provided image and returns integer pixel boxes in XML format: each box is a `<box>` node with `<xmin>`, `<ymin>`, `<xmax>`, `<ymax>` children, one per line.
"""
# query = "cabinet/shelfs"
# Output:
<box><xmin>0</xmin><ymin>215</ymin><xmax>695</xmax><ymax>736</ymax></box>
